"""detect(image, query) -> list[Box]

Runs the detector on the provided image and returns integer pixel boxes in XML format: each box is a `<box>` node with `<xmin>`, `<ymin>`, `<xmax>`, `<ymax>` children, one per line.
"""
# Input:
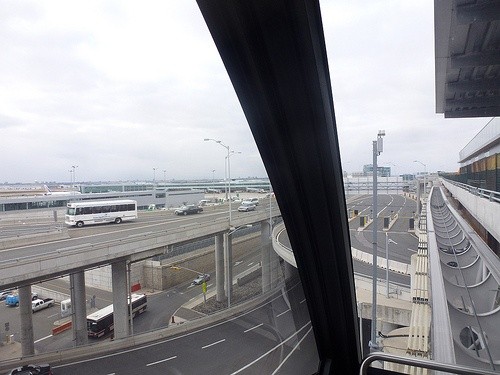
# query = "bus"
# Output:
<box><xmin>87</xmin><ymin>293</ymin><xmax>148</xmax><ymax>340</ymax></box>
<box><xmin>64</xmin><ymin>200</ymin><xmax>138</xmax><ymax>228</ymax></box>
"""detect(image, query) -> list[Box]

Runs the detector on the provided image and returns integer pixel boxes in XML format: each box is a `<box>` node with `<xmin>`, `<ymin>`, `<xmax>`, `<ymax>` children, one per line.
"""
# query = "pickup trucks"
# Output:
<box><xmin>32</xmin><ymin>298</ymin><xmax>55</xmax><ymax>315</ymax></box>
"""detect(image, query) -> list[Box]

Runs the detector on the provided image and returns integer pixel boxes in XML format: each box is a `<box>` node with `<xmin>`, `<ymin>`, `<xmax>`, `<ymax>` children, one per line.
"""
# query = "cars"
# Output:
<box><xmin>174</xmin><ymin>205</ymin><xmax>204</xmax><ymax>216</ymax></box>
<box><xmin>193</xmin><ymin>273</ymin><xmax>210</xmax><ymax>285</ymax></box>
<box><xmin>7</xmin><ymin>363</ymin><xmax>51</xmax><ymax>375</ymax></box>
<box><xmin>0</xmin><ymin>291</ymin><xmax>38</xmax><ymax>307</ymax></box>
<box><xmin>237</xmin><ymin>197</ymin><xmax>259</xmax><ymax>212</ymax></box>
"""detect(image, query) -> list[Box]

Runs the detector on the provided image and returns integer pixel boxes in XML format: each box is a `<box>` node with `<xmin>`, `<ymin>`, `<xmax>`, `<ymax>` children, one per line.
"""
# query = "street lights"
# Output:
<box><xmin>366</xmin><ymin>130</ymin><xmax>386</xmax><ymax>352</ymax></box>
<box><xmin>203</xmin><ymin>137</ymin><xmax>232</xmax><ymax>227</ymax></box>
<box><xmin>413</xmin><ymin>160</ymin><xmax>426</xmax><ymax>200</ymax></box>
<box><xmin>72</xmin><ymin>165</ymin><xmax>78</xmax><ymax>195</ymax></box>
<box><xmin>225</xmin><ymin>150</ymin><xmax>242</xmax><ymax>204</ymax></box>
<box><xmin>226</xmin><ymin>225</ymin><xmax>253</xmax><ymax>309</ymax></box>
